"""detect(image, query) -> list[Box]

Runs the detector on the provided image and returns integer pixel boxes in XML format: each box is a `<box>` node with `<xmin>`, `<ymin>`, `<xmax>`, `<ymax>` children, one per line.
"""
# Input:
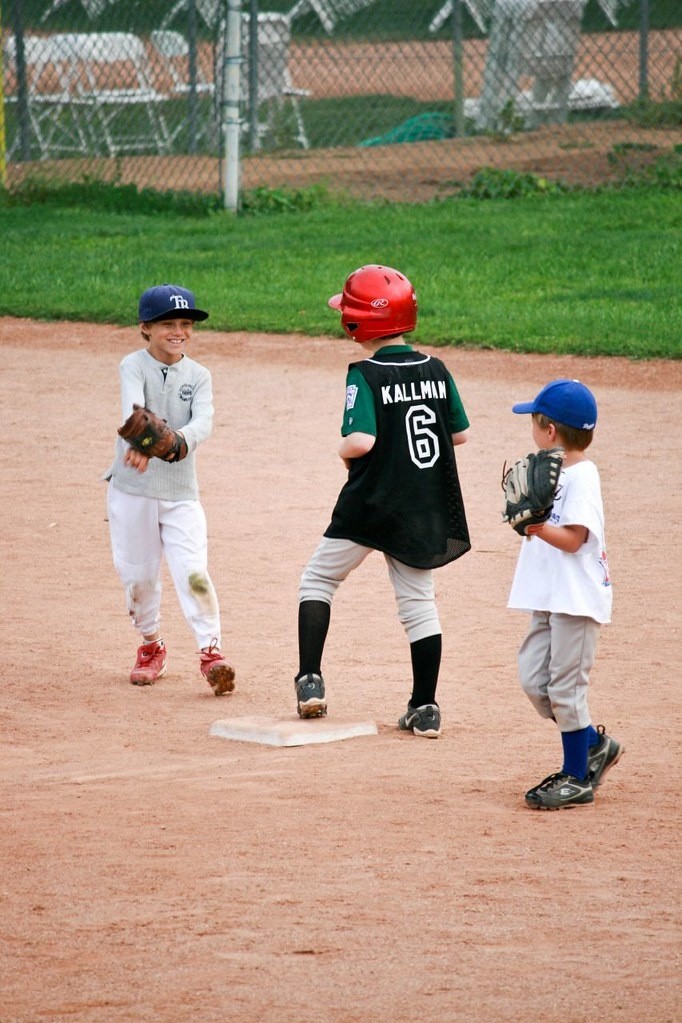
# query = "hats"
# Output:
<box><xmin>138</xmin><ymin>284</ymin><xmax>210</xmax><ymax>321</ymax></box>
<box><xmin>512</xmin><ymin>378</ymin><xmax>598</xmax><ymax>430</ymax></box>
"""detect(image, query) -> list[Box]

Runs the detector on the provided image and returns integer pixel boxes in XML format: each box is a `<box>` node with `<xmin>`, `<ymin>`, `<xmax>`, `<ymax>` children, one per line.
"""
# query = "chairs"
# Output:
<box><xmin>7</xmin><ymin>11</ymin><xmax>313</xmax><ymax>165</ymax></box>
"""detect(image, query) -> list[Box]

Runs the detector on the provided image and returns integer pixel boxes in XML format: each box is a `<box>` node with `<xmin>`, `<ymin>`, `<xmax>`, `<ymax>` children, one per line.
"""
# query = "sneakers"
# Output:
<box><xmin>586</xmin><ymin>725</ymin><xmax>626</xmax><ymax>792</ymax></box>
<box><xmin>131</xmin><ymin>643</ymin><xmax>167</xmax><ymax>684</ymax></box>
<box><xmin>525</xmin><ymin>772</ymin><xmax>595</xmax><ymax>810</ymax></box>
<box><xmin>194</xmin><ymin>637</ymin><xmax>236</xmax><ymax>696</ymax></box>
<box><xmin>295</xmin><ymin>674</ymin><xmax>327</xmax><ymax>718</ymax></box>
<box><xmin>398</xmin><ymin>700</ymin><xmax>442</xmax><ymax>737</ymax></box>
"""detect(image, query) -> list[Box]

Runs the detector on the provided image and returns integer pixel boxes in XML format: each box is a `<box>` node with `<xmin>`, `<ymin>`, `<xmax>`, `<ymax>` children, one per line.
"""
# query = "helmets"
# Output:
<box><xmin>328</xmin><ymin>264</ymin><xmax>418</xmax><ymax>343</ymax></box>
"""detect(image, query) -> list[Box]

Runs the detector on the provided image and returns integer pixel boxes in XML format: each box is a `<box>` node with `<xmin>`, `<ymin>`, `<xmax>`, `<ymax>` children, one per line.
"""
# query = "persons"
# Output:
<box><xmin>103</xmin><ymin>283</ymin><xmax>236</xmax><ymax>695</ymax></box>
<box><xmin>293</xmin><ymin>264</ymin><xmax>472</xmax><ymax>738</ymax></box>
<box><xmin>500</xmin><ymin>379</ymin><xmax>625</xmax><ymax>811</ymax></box>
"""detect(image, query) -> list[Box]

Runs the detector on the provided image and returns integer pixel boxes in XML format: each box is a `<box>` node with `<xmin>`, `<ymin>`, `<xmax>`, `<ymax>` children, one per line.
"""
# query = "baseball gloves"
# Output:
<box><xmin>115</xmin><ymin>403</ymin><xmax>188</xmax><ymax>464</ymax></box>
<box><xmin>500</xmin><ymin>447</ymin><xmax>567</xmax><ymax>541</ymax></box>
